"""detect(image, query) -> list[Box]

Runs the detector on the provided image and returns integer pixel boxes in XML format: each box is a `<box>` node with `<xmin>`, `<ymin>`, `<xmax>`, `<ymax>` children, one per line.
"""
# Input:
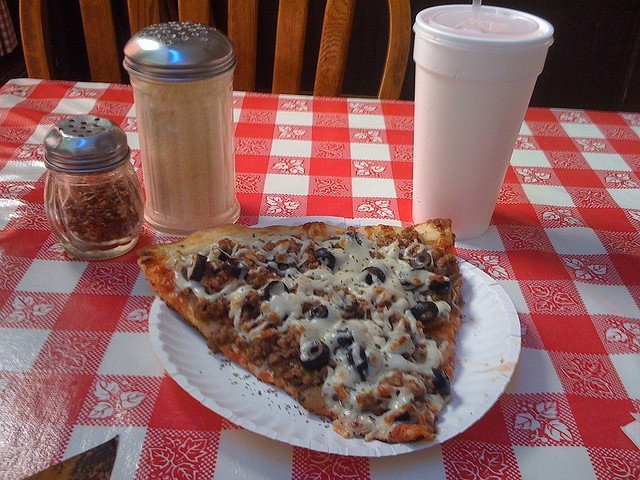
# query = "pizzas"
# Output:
<box><xmin>137</xmin><ymin>217</ymin><xmax>463</xmax><ymax>444</ymax></box>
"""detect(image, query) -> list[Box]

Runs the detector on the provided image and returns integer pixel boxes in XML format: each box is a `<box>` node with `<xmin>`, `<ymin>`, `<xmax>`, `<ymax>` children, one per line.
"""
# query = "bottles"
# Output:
<box><xmin>121</xmin><ymin>22</ymin><xmax>241</xmax><ymax>237</ymax></box>
<box><xmin>42</xmin><ymin>116</ymin><xmax>144</xmax><ymax>260</ymax></box>
<box><xmin>147</xmin><ymin>215</ymin><xmax>523</xmax><ymax>457</ymax></box>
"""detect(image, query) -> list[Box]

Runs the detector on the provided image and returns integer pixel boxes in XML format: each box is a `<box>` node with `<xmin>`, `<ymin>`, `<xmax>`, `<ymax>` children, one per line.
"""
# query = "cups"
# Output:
<box><xmin>412</xmin><ymin>5</ymin><xmax>556</xmax><ymax>235</ymax></box>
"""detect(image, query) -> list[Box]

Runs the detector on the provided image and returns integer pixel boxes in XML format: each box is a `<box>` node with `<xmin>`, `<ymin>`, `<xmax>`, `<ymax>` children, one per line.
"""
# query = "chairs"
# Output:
<box><xmin>19</xmin><ymin>1</ymin><xmax>412</xmax><ymax>99</ymax></box>
